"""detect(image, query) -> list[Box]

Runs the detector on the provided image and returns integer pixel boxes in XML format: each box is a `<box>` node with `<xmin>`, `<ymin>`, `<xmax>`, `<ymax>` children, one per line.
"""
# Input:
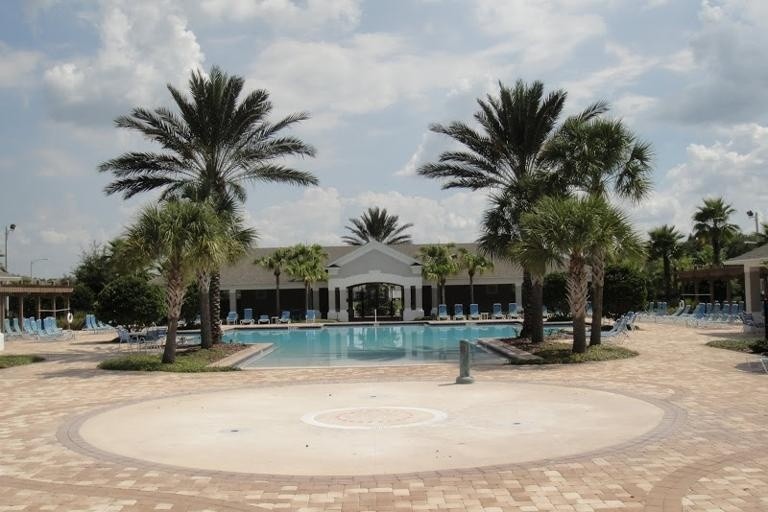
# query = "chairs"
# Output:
<box><xmin>436</xmin><ymin>304</ymin><xmax>551</xmax><ymax>321</ymax></box>
<box><xmin>586</xmin><ymin>299</ymin><xmax>766</xmax><ymax>339</ymax></box>
<box><xmin>0</xmin><ymin>313</ymin><xmax>166</xmax><ymax>352</ymax></box>
<box><xmin>227</xmin><ymin>308</ymin><xmax>318</xmax><ymax>325</ymax></box>
